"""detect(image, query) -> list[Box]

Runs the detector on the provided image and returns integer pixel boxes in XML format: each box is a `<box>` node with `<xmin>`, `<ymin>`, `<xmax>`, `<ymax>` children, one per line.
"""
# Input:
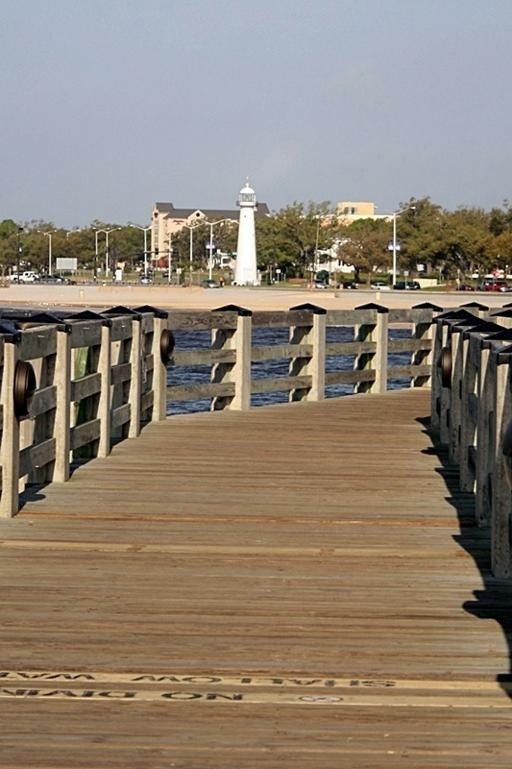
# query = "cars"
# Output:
<box><xmin>312</xmin><ymin>277</ymin><xmax>512</xmax><ymax>295</ymax></box>
<box><xmin>0</xmin><ymin>265</ymin><xmax>219</xmax><ymax>289</ymax></box>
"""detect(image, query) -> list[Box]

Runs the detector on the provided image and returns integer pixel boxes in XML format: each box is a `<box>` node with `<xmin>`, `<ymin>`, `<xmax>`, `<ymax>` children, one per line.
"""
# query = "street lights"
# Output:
<box><xmin>300</xmin><ymin>209</ymin><xmax>329</xmax><ymax>289</ymax></box>
<box><xmin>373</xmin><ymin>204</ymin><xmax>416</xmax><ymax>288</ymax></box>
<box><xmin>9</xmin><ymin>217</ymin><xmax>232</xmax><ymax>286</ymax></box>
